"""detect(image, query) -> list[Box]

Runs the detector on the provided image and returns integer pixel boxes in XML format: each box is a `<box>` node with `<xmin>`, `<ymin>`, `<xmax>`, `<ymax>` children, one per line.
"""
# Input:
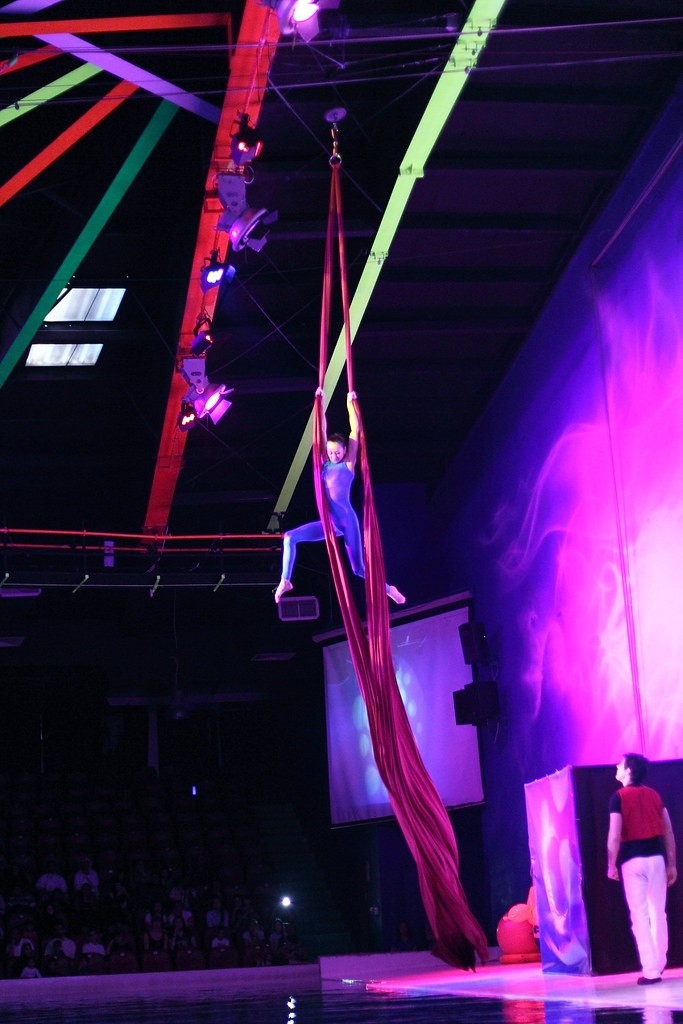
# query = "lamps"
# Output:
<box><xmin>229</xmin><ymin>114</ymin><xmax>264</xmax><ymax>165</ymax></box>
<box><xmin>260</xmin><ymin>0</ymin><xmax>338</xmax><ymax>41</ymax></box>
<box><xmin>199</xmin><ymin>250</ymin><xmax>224</xmax><ymax>294</ymax></box>
<box><xmin>175</xmin><ymin>357</ymin><xmax>234</xmax><ymax>432</ymax></box>
<box><xmin>190</xmin><ymin>332</ymin><xmax>213</xmax><ymax>357</ymax></box>
<box><xmin>213</xmin><ymin>172</ymin><xmax>279</xmax><ymax>252</ymax></box>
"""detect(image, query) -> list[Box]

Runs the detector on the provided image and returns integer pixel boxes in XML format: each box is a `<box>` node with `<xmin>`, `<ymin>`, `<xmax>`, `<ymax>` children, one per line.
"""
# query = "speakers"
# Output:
<box><xmin>459</xmin><ymin>622</ymin><xmax>490</xmax><ymax>665</ymax></box>
<box><xmin>453</xmin><ymin>680</ymin><xmax>499</xmax><ymax>725</ymax></box>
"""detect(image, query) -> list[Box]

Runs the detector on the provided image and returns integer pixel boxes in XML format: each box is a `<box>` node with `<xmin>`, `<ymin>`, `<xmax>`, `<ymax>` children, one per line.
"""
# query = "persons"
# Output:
<box><xmin>607</xmin><ymin>751</ymin><xmax>679</xmax><ymax>985</ymax></box>
<box><xmin>1</xmin><ymin>829</ymin><xmax>300</xmax><ymax>981</ymax></box>
<box><xmin>272</xmin><ymin>386</ymin><xmax>406</xmax><ymax>605</ymax></box>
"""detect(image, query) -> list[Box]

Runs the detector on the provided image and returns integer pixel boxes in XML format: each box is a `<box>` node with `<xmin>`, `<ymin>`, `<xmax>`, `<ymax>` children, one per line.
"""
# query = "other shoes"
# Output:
<box><xmin>638</xmin><ymin>977</ymin><xmax>663</xmax><ymax>984</ymax></box>
